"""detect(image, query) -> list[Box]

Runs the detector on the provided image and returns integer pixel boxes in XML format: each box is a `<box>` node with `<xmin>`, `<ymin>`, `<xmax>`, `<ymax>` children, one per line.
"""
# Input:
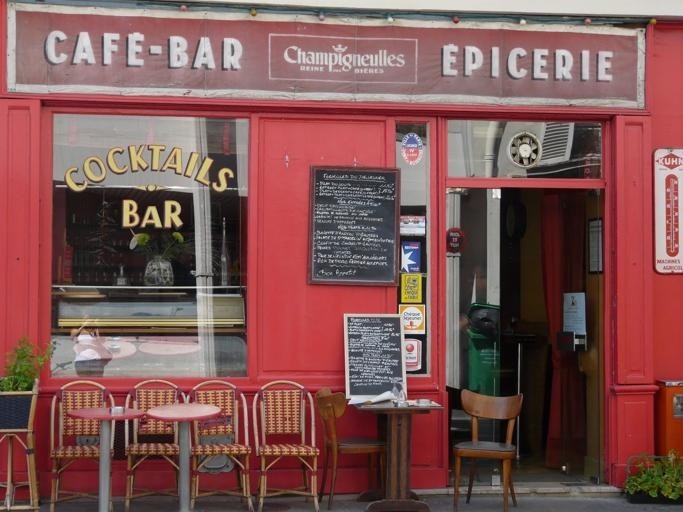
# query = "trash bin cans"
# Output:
<box><xmin>466</xmin><ymin>303</ymin><xmax>500</xmax><ymax>422</ymax></box>
<box><xmin>655</xmin><ymin>378</ymin><xmax>683</xmax><ymax>465</ymax></box>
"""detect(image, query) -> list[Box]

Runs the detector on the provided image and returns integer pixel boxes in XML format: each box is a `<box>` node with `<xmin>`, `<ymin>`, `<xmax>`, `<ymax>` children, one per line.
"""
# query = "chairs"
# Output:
<box><xmin>450</xmin><ymin>387</ymin><xmax>529</xmax><ymax>511</ymax></box>
<box><xmin>51</xmin><ymin>377</ymin><xmax>324</xmax><ymax>512</ymax></box>
<box><xmin>316</xmin><ymin>386</ymin><xmax>386</xmax><ymax>509</ymax></box>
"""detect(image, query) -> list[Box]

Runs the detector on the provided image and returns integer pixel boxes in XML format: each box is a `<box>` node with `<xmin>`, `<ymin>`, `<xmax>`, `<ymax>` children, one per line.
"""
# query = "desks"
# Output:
<box><xmin>353</xmin><ymin>396</ymin><xmax>444</xmax><ymax>511</ymax></box>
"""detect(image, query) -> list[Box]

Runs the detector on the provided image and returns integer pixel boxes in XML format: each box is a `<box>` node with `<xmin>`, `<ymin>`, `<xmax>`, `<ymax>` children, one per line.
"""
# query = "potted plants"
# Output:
<box><xmin>0</xmin><ymin>333</ymin><xmax>56</xmax><ymax>428</ymax></box>
<box><xmin>128</xmin><ymin>224</ymin><xmax>186</xmax><ymax>285</ymax></box>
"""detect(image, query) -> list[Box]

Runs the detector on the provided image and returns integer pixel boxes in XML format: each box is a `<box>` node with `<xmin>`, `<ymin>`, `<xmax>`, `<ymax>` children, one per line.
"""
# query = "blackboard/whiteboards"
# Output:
<box><xmin>343</xmin><ymin>312</ymin><xmax>409</xmax><ymax>400</ymax></box>
<box><xmin>308</xmin><ymin>165</ymin><xmax>401</xmax><ymax>286</ymax></box>
